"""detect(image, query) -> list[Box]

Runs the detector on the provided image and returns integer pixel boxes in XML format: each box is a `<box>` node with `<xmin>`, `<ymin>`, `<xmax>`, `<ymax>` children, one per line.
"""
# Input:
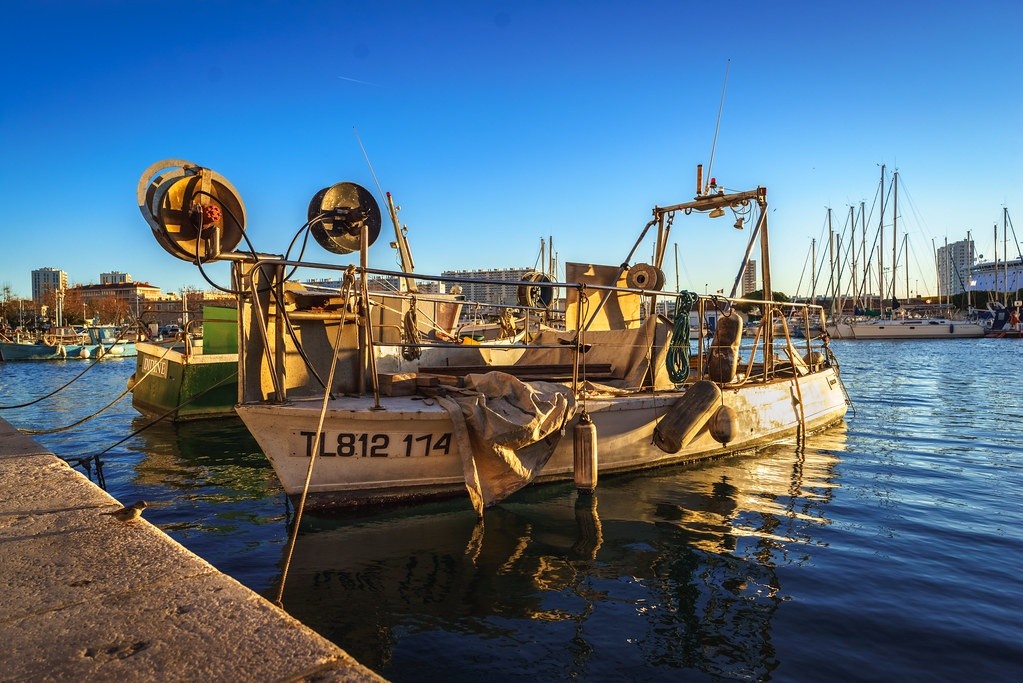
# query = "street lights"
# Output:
<box><xmin>1</xmin><ymin>293</ymin><xmax>68</xmax><ymax>327</ymax></box>
<box><xmin>181</xmin><ymin>289</ymin><xmax>188</xmax><ymax>324</ymax></box>
<box><xmin>83</xmin><ymin>302</ymin><xmax>88</xmax><ymax>322</ymax></box>
<box><xmin>136</xmin><ymin>290</ymin><xmax>140</xmax><ymax>317</ymax></box>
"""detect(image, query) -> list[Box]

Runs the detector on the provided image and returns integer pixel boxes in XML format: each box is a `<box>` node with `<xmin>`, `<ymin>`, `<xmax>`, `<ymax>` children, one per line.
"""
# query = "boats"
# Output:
<box><xmin>132</xmin><ymin>157</ymin><xmax>850</xmax><ymax>509</ymax></box>
<box><xmin>0</xmin><ymin>326</ymin><xmax>183</xmax><ymax>362</ymax></box>
<box><xmin>124</xmin><ymin>192</ymin><xmax>467</xmax><ymax>417</ymax></box>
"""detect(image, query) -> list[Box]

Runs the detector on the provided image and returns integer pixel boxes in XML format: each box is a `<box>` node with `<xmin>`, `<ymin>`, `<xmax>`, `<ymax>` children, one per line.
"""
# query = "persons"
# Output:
<box><xmin>890</xmin><ymin>295</ymin><xmax>898</xmax><ymax>320</ymax></box>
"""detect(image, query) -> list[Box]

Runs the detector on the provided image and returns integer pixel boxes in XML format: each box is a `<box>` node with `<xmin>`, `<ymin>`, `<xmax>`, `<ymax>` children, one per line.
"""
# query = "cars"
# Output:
<box><xmin>168</xmin><ymin>328</ymin><xmax>180</xmax><ymax>337</ymax></box>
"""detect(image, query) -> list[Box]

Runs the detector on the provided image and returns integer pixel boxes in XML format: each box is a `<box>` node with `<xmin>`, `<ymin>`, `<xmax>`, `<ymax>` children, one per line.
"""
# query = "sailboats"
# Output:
<box><xmin>752</xmin><ymin>163</ymin><xmax>1022</xmax><ymax>335</ymax></box>
